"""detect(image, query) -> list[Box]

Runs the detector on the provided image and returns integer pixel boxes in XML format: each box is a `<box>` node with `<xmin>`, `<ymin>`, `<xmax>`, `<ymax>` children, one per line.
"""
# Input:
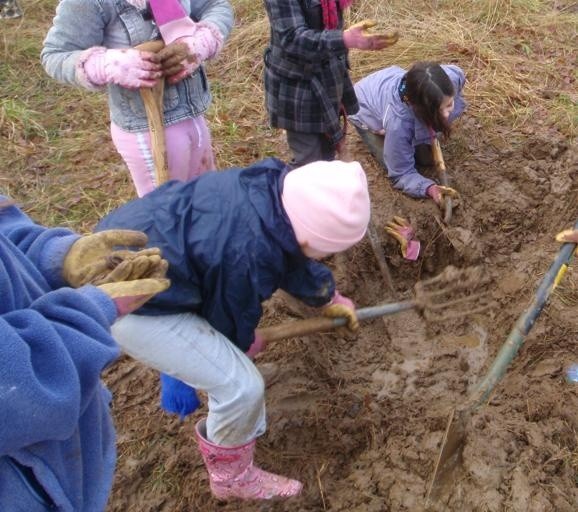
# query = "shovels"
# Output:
<box><xmin>428</xmin><ymin>228</ymin><xmax>577</xmax><ymax>499</ymax></box>
<box><xmin>431</xmin><ymin>132</ymin><xmax>484</xmax><ymax>258</ymax></box>
<box><xmin>336</xmin><ymin>140</ymin><xmax>418</xmax><ymax>346</ymax></box>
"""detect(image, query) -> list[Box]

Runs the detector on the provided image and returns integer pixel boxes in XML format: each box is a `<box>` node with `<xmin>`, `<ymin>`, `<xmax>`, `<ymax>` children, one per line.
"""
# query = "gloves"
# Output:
<box><xmin>57</xmin><ymin>228</ymin><xmax>172</xmax><ymax>321</ymax></box>
<box><xmin>74</xmin><ymin>44</ymin><xmax>163</xmax><ymax>91</ymax></box>
<box><xmin>425</xmin><ymin>182</ymin><xmax>461</xmax><ymax>211</ymax></box>
<box><xmin>551</xmin><ymin>225</ymin><xmax>578</xmax><ymax>254</ymax></box>
<box><xmin>341</xmin><ymin>17</ymin><xmax>400</xmax><ymax>53</ymax></box>
<box><xmin>312</xmin><ymin>289</ymin><xmax>361</xmax><ymax>340</ymax></box>
<box><xmin>148</xmin><ymin>18</ymin><xmax>224</xmax><ymax>88</ymax></box>
<box><xmin>247</xmin><ymin>330</ymin><xmax>267</xmax><ymax>358</ymax></box>
<box><xmin>382</xmin><ymin>214</ymin><xmax>421</xmax><ymax>262</ymax></box>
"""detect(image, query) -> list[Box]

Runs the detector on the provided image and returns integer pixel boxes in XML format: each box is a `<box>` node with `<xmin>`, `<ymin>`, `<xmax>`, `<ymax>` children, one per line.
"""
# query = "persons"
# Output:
<box><xmin>39</xmin><ymin>1</ymin><xmax>236</xmax><ymax>203</ymax></box>
<box><xmin>347</xmin><ymin>58</ymin><xmax>473</xmax><ymax>212</ymax></box>
<box><xmin>89</xmin><ymin>159</ymin><xmax>372</xmax><ymax>501</ymax></box>
<box><xmin>0</xmin><ymin>193</ymin><xmax>172</xmax><ymax>511</ymax></box>
<box><xmin>260</xmin><ymin>0</ymin><xmax>399</xmax><ymax>168</ymax></box>
<box><xmin>555</xmin><ymin>226</ymin><xmax>578</xmax><ymax>387</ymax></box>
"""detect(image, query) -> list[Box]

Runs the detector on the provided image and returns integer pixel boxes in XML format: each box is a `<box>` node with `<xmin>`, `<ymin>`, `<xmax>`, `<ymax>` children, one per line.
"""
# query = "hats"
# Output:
<box><xmin>277</xmin><ymin>157</ymin><xmax>372</xmax><ymax>256</ymax></box>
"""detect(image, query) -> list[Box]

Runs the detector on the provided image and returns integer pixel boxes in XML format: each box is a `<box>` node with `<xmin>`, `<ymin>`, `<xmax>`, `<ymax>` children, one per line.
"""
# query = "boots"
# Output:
<box><xmin>191</xmin><ymin>415</ymin><xmax>304</xmax><ymax>499</ymax></box>
